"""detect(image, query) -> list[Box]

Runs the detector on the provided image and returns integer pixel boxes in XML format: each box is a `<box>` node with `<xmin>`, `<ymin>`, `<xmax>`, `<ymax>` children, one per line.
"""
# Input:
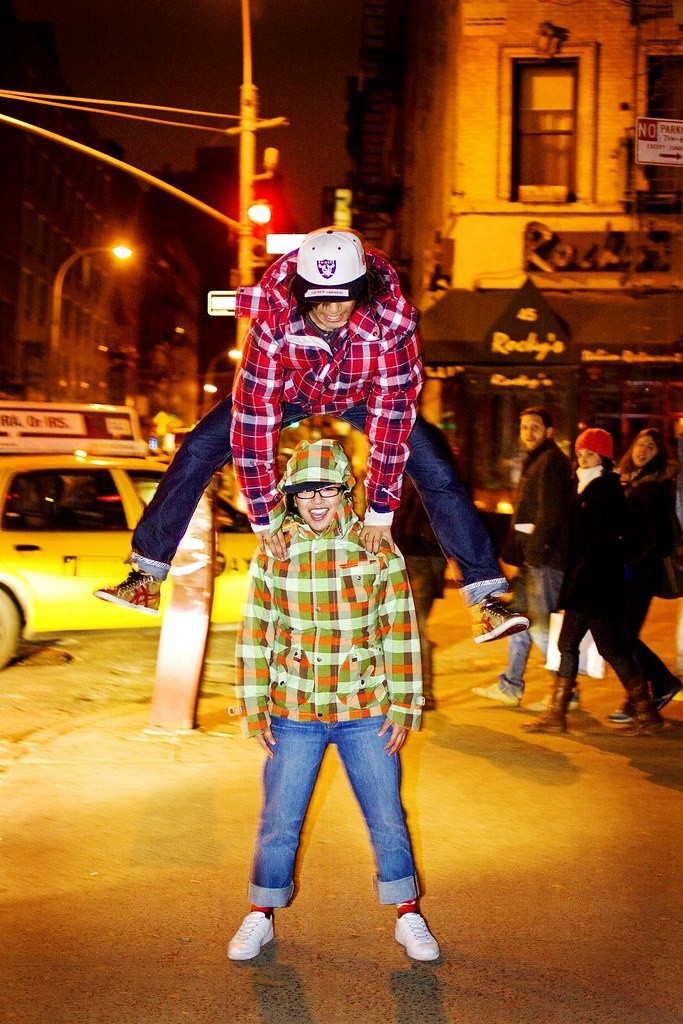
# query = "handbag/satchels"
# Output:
<box><xmin>654</xmin><ymin>557</ymin><xmax>683</xmax><ymax>599</ymax></box>
<box><xmin>545</xmin><ymin>614</ymin><xmax>605</xmax><ymax>678</ymax></box>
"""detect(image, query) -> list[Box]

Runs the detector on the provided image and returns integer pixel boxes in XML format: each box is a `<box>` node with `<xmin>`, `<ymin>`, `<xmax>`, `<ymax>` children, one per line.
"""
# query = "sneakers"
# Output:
<box><xmin>394</xmin><ymin>913</ymin><xmax>439</xmax><ymax>962</ymax></box>
<box><xmin>657</xmin><ymin>676</ymin><xmax>683</xmax><ymax>711</ymax></box>
<box><xmin>227</xmin><ymin>911</ymin><xmax>273</xmax><ymax>960</ymax></box>
<box><xmin>608</xmin><ymin>713</ymin><xmax>632</xmax><ymax>723</ymax></box>
<box><xmin>93</xmin><ymin>568</ymin><xmax>160</xmax><ymax>614</ymax></box>
<box><xmin>525</xmin><ymin>690</ymin><xmax>580</xmax><ymax>712</ymax></box>
<box><xmin>472</xmin><ymin>681</ymin><xmax>522</xmax><ymax>706</ymax></box>
<box><xmin>470</xmin><ymin>596</ymin><xmax>530</xmax><ymax>644</ymax></box>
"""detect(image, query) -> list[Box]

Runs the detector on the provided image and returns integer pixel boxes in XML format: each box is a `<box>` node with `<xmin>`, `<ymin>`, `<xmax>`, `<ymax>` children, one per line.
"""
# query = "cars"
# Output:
<box><xmin>0</xmin><ymin>398</ymin><xmax>258</xmax><ymax>672</ymax></box>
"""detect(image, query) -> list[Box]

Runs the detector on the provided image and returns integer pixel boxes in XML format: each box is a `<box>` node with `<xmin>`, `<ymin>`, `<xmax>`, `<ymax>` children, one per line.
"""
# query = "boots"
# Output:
<box><xmin>523</xmin><ymin>677</ymin><xmax>574</xmax><ymax>732</ymax></box>
<box><xmin>617</xmin><ymin>683</ymin><xmax>664</xmax><ymax>736</ymax></box>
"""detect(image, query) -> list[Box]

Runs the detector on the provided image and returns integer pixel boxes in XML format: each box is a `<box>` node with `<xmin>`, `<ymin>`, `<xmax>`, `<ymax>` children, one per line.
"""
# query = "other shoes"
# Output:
<box><xmin>422</xmin><ymin>699</ymin><xmax>434</xmax><ymax>711</ymax></box>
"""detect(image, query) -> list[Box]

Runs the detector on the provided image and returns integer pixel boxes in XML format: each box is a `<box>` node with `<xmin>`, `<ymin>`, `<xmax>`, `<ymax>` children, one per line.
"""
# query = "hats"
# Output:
<box><xmin>575</xmin><ymin>428</ymin><xmax>613</xmax><ymax>459</ymax></box>
<box><xmin>283</xmin><ymin>482</ymin><xmax>330</xmax><ymax>495</ymax></box>
<box><xmin>294</xmin><ymin>226</ymin><xmax>368</xmax><ymax>302</ymax></box>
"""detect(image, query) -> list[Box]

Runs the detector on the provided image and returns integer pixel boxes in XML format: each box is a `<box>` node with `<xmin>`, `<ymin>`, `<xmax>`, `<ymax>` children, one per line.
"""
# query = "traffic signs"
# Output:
<box><xmin>635</xmin><ymin>116</ymin><xmax>683</xmax><ymax>168</ymax></box>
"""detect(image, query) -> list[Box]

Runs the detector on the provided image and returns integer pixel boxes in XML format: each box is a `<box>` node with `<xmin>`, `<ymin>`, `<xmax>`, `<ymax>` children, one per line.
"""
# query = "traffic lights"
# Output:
<box><xmin>247</xmin><ymin>202</ymin><xmax>274</xmax><ymax>268</ymax></box>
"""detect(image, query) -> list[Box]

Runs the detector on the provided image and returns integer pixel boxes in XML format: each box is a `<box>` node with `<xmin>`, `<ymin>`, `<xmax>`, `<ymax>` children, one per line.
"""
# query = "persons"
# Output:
<box><xmin>519</xmin><ymin>428</ymin><xmax>657</xmax><ymax>733</ymax></box>
<box><xmin>224</xmin><ymin>439</ymin><xmax>440</xmax><ymax>962</ymax></box>
<box><xmin>473</xmin><ymin>407</ymin><xmax>582</xmax><ymax>712</ymax></box>
<box><xmin>606</xmin><ymin>426</ymin><xmax>683</xmax><ymax>722</ymax></box>
<box><xmin>91</xmin><ymin>226</ymin><xmax>532</xmax><ymax>645</ymax></box>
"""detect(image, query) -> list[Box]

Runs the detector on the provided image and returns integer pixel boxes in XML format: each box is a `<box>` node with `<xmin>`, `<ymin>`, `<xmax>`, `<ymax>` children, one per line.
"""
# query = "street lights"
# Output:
<box><xmin>46</xmin><ymin>238</ymin><xmax>133</xmax><ymax>402</ymax></box>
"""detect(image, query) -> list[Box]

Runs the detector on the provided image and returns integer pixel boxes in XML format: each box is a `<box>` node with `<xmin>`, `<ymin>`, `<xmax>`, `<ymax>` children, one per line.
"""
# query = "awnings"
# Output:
<box><xmin>420</xmin><ymin>290</ymin><xmax>683</xmax><ymax>391</ymax></box>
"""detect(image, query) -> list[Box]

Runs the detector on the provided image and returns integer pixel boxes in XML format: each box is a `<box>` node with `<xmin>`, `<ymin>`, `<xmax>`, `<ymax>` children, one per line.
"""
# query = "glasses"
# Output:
<box><xmin>295</xmin><ymin>486</ymin><xmax>344</xmax><ymax>499</ymax></box>
